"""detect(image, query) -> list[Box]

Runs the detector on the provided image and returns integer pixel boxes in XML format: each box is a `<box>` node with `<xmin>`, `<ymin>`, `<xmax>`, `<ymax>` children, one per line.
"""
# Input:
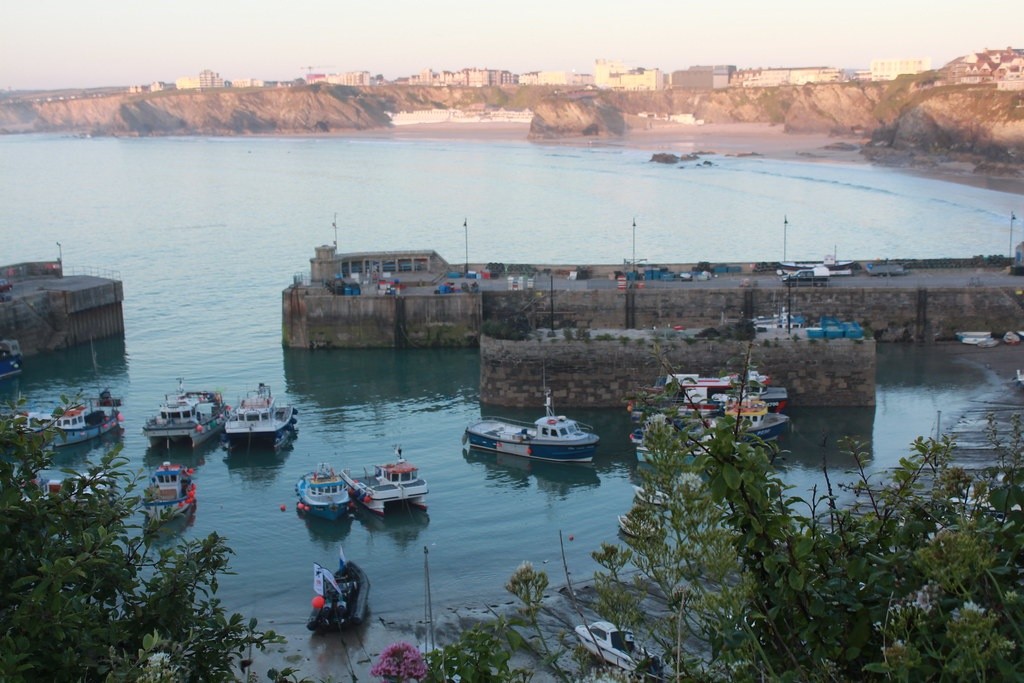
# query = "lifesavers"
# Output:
<box><xmin>547</xmin><ymin>419</ymin><xmax>557</xmax><ymax>424</ymax></box>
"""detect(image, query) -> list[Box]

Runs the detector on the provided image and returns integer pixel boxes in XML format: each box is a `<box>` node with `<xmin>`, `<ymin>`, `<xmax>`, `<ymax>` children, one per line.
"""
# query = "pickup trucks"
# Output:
<box><xmin>780</xmin><ymin>269</ymin><xmax>831</xmax><ymax>287</ymax></box>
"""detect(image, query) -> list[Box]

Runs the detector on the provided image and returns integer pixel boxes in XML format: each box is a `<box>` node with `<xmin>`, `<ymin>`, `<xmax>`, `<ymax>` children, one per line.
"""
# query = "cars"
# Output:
<box><xmin>0</xmin><ymin>279</ymin><xmax>13</xmax><ymax>292</ymax></box>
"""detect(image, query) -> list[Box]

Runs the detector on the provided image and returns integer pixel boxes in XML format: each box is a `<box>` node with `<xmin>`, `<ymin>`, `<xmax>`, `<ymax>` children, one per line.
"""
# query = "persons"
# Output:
<box><xmin>181</xmin><ymin>480</ymin><xmax>188</xmax><ymax>496</ymax></box>
<box><xmin>704</xmin><ymin>419</ymin><xmax>711</xmax><ymax>428</ymax></box>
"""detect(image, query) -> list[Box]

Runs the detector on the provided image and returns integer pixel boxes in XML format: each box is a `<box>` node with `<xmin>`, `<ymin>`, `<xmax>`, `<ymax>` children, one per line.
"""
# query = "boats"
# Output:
<box><xmin>137</xmin><ymin>460</ymin><xmax>197</xmax><ymax>522</ymax></box>
<box><xmin>292</xmin><ymin>463</ymin><xmax>356</xmax><ymax>522</ymax></box>
<box><xmin>617</xmin><ymin>512</ymin><xmax>657</xmax><ymax>540</ymax></box>
<box><xmin>976</xmin><ymin>339</ymin><xmax>1001</xmax><ymax>348</ymax></box>
<box><xmin>631</xmin><ymin>483</ymin><xmax>675</xmax><ymax>508</ymax></box>
<box><xmin>337</xmin><ymin>443</ymin><xmax>430</xmax><ymax>517</ymax></box>
<box><xmin>12</xmin><ymin>390</ymin><xmax>126</xmax><ymax>448</ymax></box>
<box><xmin>459</xmin><ymin>356</ymin><xmax>601</xmax><ymax>464</ymax></box>
<box><xmin>575</xmin><ymin>620</ymin><xmax>677</xmax><ymax>683</ymax></box>
<box><xmin>24</xmin><ymin>467</ymin><xmax>116</xmax><ymax>526</ymax></box>
<box><xmin>305</xmin><ymin>544</ymin><xmax>370</xmax><ymax>635</ymax></box>
<box><xmin>955</xmin><ymin>330</ymin><xmax>992</xmax><ymax>344</ymax></box>
<box><xmin>0</xmin><ymin>339</ymin><xmax>24</xmax><ymax>380</ymax></box>
<box><xmin>140</xmin><ymin>377</ymin><xmax>231</xmax><ymax>448</ymax></box>
<box><xmin>625</xmin><ymin>360</ymin><xmax>792</xmax><ymax>466</ymax></box>
<box><xmin>222</xmin><ymin>382</ymin><xmax>298</xmax><ymax>450</ymax></box>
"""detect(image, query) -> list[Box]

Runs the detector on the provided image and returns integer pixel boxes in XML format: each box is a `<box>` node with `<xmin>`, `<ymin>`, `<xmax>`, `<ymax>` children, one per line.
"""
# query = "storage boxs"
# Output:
<box><xmin>344</xmin><ymin>287</ymin><xmax>361</xmax><ymax>296</ymax></box>
<box><xmin>804</xmin><ymin>315</ymin><xmax>864</xmax><ymax>340</ymax></box>
<box><xmin>625</xmin><ymin>265</ymin><xmax>742</xmax><ymax>281</ymax></box>
<box><xmin>439</xmin><ymin>285</ymin><xmax>451</xmax><ymax>295</ymax></box>
